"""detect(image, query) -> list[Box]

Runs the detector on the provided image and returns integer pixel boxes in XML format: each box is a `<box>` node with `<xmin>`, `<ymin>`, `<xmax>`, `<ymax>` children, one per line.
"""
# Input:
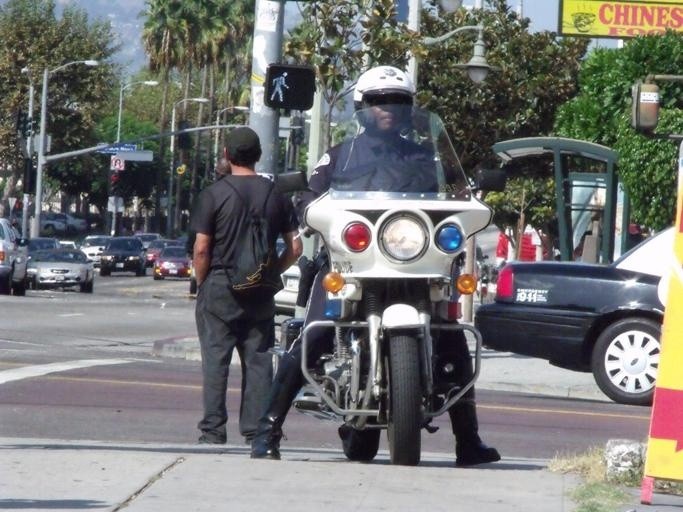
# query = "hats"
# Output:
<box><xmin>226</xmin><ymin>124</ymin><xmax>260</xmax><ymax>149</ymax></box>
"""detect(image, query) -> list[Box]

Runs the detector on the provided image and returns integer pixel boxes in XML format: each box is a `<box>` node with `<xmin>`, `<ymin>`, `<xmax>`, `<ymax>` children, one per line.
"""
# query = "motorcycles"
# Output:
<box><xmin>263</xmin><ymin>103</ymin><xmax>514</xmax><ymax>467</ymax></box>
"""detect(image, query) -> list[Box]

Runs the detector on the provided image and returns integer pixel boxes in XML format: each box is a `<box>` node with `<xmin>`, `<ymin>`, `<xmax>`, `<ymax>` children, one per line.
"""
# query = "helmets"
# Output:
<box><xmin>351</xmin><ymin>64</ymin><xmax>417</xmax><ymax>135</ymax></box>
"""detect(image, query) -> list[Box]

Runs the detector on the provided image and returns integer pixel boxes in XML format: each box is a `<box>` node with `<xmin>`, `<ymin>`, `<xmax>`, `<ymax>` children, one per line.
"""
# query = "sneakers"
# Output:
<box><xmin>198</xmin><ymin>433</ymin><xmax>227</xmax><ymax>445</ymax></box>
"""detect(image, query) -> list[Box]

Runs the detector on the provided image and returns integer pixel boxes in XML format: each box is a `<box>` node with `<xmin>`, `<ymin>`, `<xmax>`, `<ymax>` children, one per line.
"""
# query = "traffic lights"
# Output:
<box><xmin>631</xmin><ymin>84</ymin><xmax>657</xmax><ymax>129</ymax></box>
<box><xmin>264</xmin><ymin>65</ymin><xmax>315</xmax><ymax>112</ymax></box>
<box><xmin>110</xmin><ymin>172</ymin><xmax>119</xmax><ymax>196</ymax></box>
<box><xmin>18</xmin><ymin>117</ymin><xmax>25</xmax><ymax>134</ymax></box>
<box><xmin>23</xmin><ymin>118</ymin><xmax>32</xmax><ymax>140</ymax></box>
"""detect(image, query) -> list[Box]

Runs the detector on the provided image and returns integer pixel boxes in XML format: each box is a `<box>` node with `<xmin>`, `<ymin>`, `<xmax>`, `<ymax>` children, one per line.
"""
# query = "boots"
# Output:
<box><xmin>250</xmin><ymin>350</ymin><xmax>309</xmax><ymax>460</ymax></box>
<box><xmin>445</xmin><ymin>360</ymin><xmax>501</xmax><ymax>466</ymax></box>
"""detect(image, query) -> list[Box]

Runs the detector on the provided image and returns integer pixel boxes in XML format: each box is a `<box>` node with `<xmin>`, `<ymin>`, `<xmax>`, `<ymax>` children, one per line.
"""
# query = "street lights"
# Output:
<box><xmin>212</xmin><ymin>104</ymin><xmax>249</xmax><ymax>178</ymax></box>
<box><xmin>283</xmin><ymin>116</ymin><xmax>336</xmax><ymax>170</ymax></box>
<box><xmin>32</xmin><ymin>59</ymin><xmax>102</xmax><ymax>237</ymax></box>
<box><xmin>164</xmin><ymin>95</ymin><xmax>211</xmax><ymax>239</ymax></box>
<box><xmin>397</xmin><ymin>20</ymin><xmax>492</xmax><ymax>111</ymax></box>
<box><xmin>20</xmin><ymin>67</ymin><xmax>35</xmax><ymax>241</ymax></box>
<box><xmin>110</xmin><ymin>77</ymin><xmax>161</xmax><ymax>236</ymax></box>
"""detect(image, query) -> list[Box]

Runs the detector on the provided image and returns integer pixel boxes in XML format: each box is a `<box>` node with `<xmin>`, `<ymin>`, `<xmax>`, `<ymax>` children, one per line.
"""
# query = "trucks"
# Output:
<box><xmin>491</xmin><ymin>220</ymin><xmax>553</xmax><ymax>290</ymax></box>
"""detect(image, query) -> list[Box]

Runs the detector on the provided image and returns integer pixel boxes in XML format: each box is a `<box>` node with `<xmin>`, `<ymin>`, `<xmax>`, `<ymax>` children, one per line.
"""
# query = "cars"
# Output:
<box><xmin>0</xmin><ymin>218</ymin><xmax>30</xmax><ymax>297</ymax></box>
<box><xmin>34</xmin><ymin>247</ymin><xmax>97</xmax><ymax>295</ymax></box>
<box><xmin>0</xmin><ymin>203</ymin><xmax>193</xmax><ymax>279</ymax></box>
<box><xmin>474</xmin><ymin>246</ymin><xmax>489</xmax><ymax>292</ymax></box>
<box><xmin>269</xmin><ymin>237</ymin><xmax>301</xmax><ymax>310</ymax></box>
<box><xmin>469</xmin><ymin>209</ymin><xmax>678</xmax><ymax>411</ymax></box>
<box><xmin>24</xmin><ymin>248</ymin><xmax>45</xmax><ymax>290</ymax></box>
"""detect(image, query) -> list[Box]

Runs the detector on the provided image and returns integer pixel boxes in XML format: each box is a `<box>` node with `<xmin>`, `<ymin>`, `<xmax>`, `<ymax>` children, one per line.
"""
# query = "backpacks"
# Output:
<box><xmin>221</xmin><ymin>176</ymin><xmax>279</xmax><ymax>300</ymax></box>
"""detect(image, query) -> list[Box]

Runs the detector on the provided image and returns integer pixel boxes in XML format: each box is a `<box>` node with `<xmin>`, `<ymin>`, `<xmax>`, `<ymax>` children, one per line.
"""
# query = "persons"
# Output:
<box><xmin>250</xmin><ymin>65</ymin><xmax>501</xmax><ymax>464</ymax></box>
<box><xmin>193</xmin><ymin>129</ymin><xmax>303</xmax><ymax>444</ymax></box>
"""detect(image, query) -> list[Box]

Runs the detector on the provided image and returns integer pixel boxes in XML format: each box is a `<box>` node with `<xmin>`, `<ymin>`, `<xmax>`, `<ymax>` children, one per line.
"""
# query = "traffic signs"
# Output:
<box><xmin>96</xmin><ymin>141</ymin><xmax>137</xmax><ymax>154</ymax></box>
